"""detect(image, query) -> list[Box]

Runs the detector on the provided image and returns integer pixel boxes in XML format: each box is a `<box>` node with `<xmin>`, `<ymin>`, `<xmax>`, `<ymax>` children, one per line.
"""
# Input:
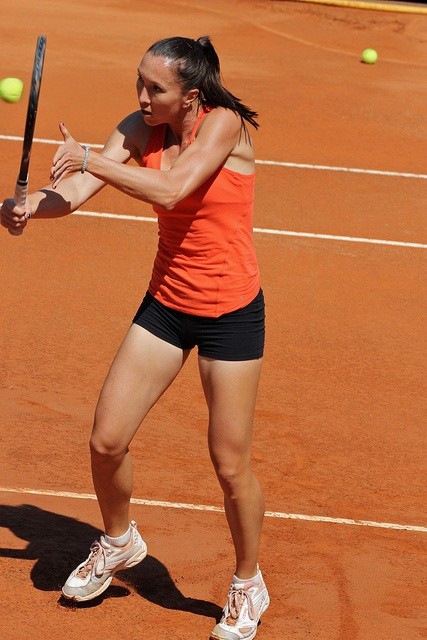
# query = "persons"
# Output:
<box><xmin>0</xmin><ymin>35</ymin><xmax>265</xmax><ymax>635</ymax></box>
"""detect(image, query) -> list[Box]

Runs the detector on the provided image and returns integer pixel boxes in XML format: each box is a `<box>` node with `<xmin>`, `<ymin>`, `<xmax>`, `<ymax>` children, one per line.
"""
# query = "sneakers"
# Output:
<box><xmin>210</xmin><ymin>563</ymin><xmax>270</xmax><ymax>639</ymax></box>
<box><xmin>62</xmin><ymin>519</ymin><xmax>148</xmax><ymax>602</ymax></box>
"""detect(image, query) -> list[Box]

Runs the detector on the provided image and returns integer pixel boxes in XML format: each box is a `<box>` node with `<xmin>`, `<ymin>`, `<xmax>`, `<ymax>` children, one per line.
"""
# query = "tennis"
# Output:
<box><xmin>361</xmin><ymin>49</ymin><xmax>378</xmax><ymax>64</ymax></box>
<box><xmin>0</xmin><ymin>77</ymin><xmax>23</xmax><ymax>103</ymax></box>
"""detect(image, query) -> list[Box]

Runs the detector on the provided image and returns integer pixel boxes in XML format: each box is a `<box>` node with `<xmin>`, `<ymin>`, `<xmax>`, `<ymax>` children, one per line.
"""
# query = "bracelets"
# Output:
<box><xmin>77</xmin><ymin>141</ymin><xmax>92</xmax><ymax>174</ymax></box>
